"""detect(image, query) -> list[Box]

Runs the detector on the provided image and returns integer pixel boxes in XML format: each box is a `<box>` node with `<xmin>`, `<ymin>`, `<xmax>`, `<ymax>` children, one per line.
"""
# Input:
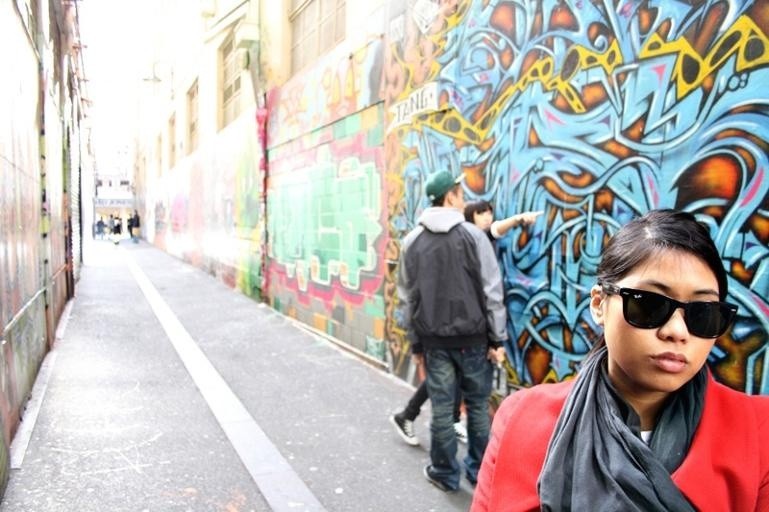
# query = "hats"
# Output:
<box><xmin>422</xmin><ymin>167</ymin><xmax>467</xmax><ymax>204</ymax></box>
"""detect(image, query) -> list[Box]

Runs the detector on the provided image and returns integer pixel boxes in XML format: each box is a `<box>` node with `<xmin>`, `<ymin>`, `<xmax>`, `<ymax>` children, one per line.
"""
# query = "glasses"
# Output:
<box><xmin>601</xmin><ymin>281</ymin><xmax>740</xmax><ymax>339</ymax></box>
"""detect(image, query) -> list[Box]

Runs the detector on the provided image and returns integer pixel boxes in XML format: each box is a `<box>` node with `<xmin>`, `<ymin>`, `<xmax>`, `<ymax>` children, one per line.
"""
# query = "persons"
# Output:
<box><xmin>467</xmin><ymin>208</ymin><xmax>769</xmax><ymax>512</ymax></box>
<box><xmin>396</xmin><ymin>168</ymin><xmax>510</xmax><ymax>496</ymax></box>
<box><xmin>94</xmin><ymin>208</ymin><xmax>140</xmax><ymax>246</ymax></box>
<box><xmin>390</xmin><ymin>197</ymin><xmax>547</xmax><ymax>451</ymax></box>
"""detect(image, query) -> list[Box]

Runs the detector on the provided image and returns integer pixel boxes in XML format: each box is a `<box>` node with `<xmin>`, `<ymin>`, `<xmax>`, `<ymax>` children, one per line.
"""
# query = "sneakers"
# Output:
<box><xmin>388</xmin><ymin>411</ymin><xmax>420</xmax><ymax>447</ymax></box>
<box><xmin>423</xmin><ymin>463</ymin><xmax>459</xmax><ymax>495</ymax></box>
<box><xmin>453</xmin><ymin>420</ymin><xmax>469</xmax><ymax>445</ymax></box>
